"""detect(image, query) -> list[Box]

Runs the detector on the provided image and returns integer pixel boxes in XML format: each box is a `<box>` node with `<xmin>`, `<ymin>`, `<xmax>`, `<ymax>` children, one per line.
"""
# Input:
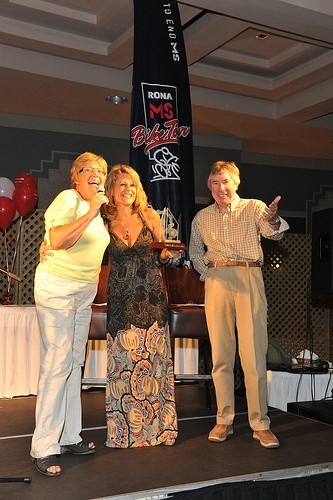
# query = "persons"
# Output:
<box><xmin>38</xmin><ymin>165</ymin><xmax>185</xmax><ymax>448</ymax></box>
<box><xmin>189</xmin><ymin>160</ymin><xmax>289</xmax><ymax>449</ymax></box>
<box><xmin>29</xmin><ymin>152</ymin><xmax>110</xmax><ymax>481</ymax></box>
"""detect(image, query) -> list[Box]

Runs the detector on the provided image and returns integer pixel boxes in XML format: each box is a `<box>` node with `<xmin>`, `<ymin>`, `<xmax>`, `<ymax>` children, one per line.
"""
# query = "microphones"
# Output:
<box><xmin>97</xmin><ymin>185</ymin><xmax>107</xmax><ymax>219</ymax></box>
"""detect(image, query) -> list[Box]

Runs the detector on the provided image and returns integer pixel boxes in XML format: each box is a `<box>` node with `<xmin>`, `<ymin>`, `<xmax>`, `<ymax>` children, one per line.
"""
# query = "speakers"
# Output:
<box><xmin>309</xmin><ymin>207</ymin><xmax>332</xmax><ymax>309</ymax></box>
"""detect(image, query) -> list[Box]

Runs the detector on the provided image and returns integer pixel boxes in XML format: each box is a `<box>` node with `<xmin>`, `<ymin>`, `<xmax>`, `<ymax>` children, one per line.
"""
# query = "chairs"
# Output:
<box><xmin>81</xmin><ymin>259</ymin><xmax>212</xmax><ymax>388</ymax></box>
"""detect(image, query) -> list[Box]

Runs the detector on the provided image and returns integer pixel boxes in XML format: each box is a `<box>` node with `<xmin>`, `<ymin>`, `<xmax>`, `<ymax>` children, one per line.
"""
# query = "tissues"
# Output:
<box><xmin>295</xmin><ymin>349</ymin><xmax>319</xmax><ymax>366</ymax></box>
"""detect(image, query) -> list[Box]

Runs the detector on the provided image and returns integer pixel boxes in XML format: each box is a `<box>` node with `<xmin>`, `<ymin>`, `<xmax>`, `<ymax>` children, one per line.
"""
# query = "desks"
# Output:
<box><xmin>267</xmin><ymin>340</ymin><xmax>333</xmax><ymax>413</ymax></box>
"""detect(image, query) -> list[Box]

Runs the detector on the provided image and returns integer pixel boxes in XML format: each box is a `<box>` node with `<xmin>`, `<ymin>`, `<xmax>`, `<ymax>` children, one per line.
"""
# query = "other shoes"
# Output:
<box><xmin>208</xmin><ymin>424</ymin><xmax>234</xmax><ymax>442</ymax></box>
<box><xmin>161</xmin><ymin>438</ymin><xmax>175</xmax><ymax>445</ymax></box>
<box><xmin>252</xmin><ymin>429</ymin><xmax>279</xmax><ymax>449</ymax></box>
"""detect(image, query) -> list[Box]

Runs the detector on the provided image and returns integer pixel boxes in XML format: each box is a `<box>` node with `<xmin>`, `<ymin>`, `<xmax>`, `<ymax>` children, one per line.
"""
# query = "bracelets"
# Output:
<box><xmin>268</xmin><ymin>216</ymin><xmax>280</xmax><ymax>225</ymax></box>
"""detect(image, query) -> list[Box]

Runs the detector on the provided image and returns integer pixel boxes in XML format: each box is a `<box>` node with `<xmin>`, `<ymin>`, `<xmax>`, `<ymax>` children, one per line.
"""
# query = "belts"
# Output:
<box><xmin>207</xmin><ymin>261</ymin><xmax>261</xmax><ymax>268</ymax></box>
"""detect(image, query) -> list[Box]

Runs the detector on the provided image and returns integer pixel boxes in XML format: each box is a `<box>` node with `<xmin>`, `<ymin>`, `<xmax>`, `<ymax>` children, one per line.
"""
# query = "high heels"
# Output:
<box><xmin>59</xmin><ymin>440</ymin><xmax>96</xmax><ymax>455</ymax></box>
<box><xmin>31</xmin><ymin>454</ymin><xmax>64</xmax><ymax>477</ymax></box>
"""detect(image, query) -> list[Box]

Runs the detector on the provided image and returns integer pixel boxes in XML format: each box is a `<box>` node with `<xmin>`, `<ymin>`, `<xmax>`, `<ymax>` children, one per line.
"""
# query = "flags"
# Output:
<box><xmin>128</xmin><ymin>0</ymin><xmax>198</xmax><ymax>269</ymax></box>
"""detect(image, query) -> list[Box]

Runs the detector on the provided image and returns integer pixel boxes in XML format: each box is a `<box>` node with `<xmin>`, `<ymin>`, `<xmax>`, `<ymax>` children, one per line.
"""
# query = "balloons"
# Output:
<box><xmin>0</xmin><ymin>173</ymin><xmax>38</xmax><ymax>230</ymax></box>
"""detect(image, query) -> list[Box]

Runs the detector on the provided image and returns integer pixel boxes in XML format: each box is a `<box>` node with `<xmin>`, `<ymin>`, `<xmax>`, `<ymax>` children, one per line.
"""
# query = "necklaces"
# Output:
<box><xmin>116</xmin><ymin>211</ymin><xmax>133</xmax><ymax>240</ymax></box>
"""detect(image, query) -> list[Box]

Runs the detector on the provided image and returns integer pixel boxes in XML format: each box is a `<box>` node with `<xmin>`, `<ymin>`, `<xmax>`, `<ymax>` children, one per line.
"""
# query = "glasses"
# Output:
<box><xmin>77</xmin><ymin>167</ymin><xmax>107</xmax><ymax>177</ymax></box>
